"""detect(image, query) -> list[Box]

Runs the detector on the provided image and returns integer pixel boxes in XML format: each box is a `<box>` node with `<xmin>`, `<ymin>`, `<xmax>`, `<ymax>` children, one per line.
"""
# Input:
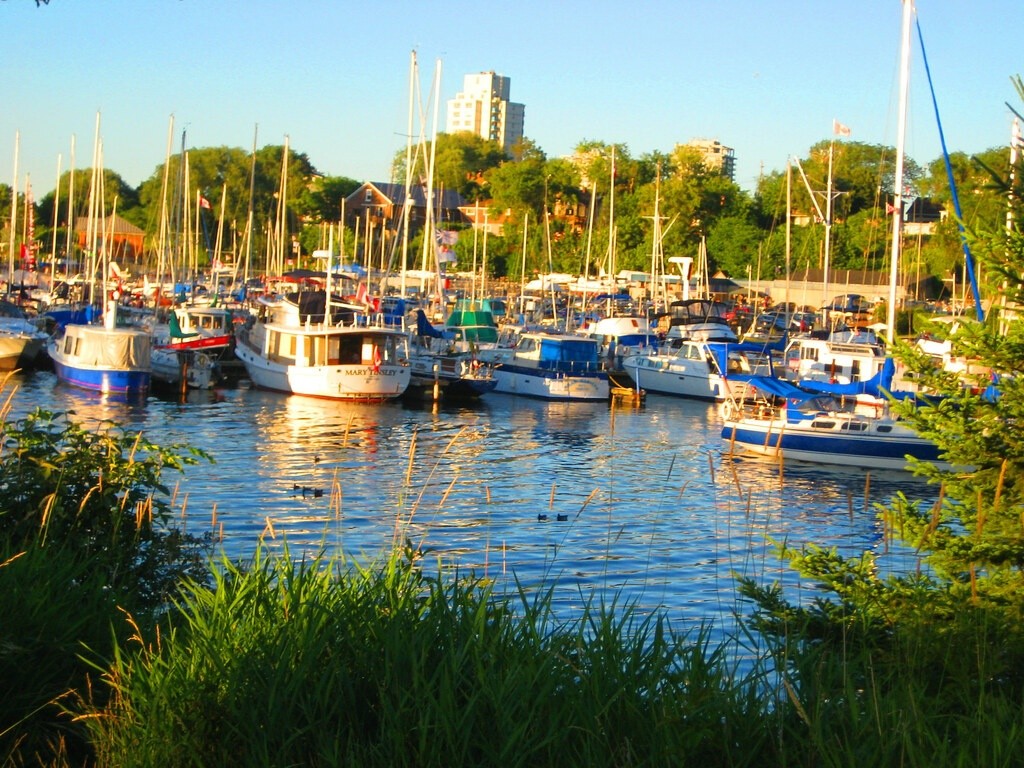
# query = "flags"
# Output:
<box><xmin>199</xmin><ymin>196</ymin><xmax>210</xmax><ymax>209</ymax></box>
<box><xmin>356</xmin><ymin>282</ymin><xmax>381</xmax><ymax>311</ymax></box>
<box><xmin>439</xmin><ymin>246</ymin><xmax>456</xmax><ymax>263</ymax></box>
<box><xmin>887</xmin><ymin>203</ymin><xmax>896</xmax><ymax>213</ymax></box>
<box><xmin>436</xmin><ymin>230</ymin><xmax>458</xmax><ymax>244</ymax></box>
<box><xmin>834</xmin><ymin>120</ymin><xmax>851</xmax><ymax>137</ymax></box>
<box><xmin>20</xmin><ymin>244</ymin><xmax>27</xmax><ymax>258</ymax></box>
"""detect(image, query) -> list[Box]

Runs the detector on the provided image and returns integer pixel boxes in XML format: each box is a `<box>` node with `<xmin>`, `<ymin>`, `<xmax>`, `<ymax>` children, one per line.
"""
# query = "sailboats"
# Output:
<box><xmin>1</xmin><ymin>49</ymin><xmax>1024</xmax><ymax>403</ymax></box>
<box><xmin>720</xmin><ymin>0</ymin><xmax>1003</xmax><ymax>474</ymax></box>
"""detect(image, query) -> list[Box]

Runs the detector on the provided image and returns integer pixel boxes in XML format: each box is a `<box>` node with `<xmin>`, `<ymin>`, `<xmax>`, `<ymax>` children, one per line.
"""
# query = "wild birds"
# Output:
<box><xmin>557</xmin><ymin>514</ymin><xmax>568</xmax><ymax>521</ymax></box>
<box><xmin>315</xmin><ymin>456</ymin><xmax>320</xmax><ymax>462</ymax></box>
<box><xmin>293</xmin><ymin>483</ymin><xmax>324</xmax><ymax>494</ymax></box>
<box><xmin>537</xmin><ymin>513</ymin><xmax>546</xmax><ymax>520</ymax></box>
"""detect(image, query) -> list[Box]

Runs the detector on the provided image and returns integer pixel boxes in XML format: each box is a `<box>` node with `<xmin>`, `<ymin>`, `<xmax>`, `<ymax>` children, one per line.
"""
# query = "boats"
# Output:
<box><xmin>622</xmin><ymin>340</ymin><xmax>774</xmax><ymax>401</ymax></box>
<box><xmin>235</xmin><ymin>222</ymin><xmax>413</xmax><ymax>402</ymax></box>
